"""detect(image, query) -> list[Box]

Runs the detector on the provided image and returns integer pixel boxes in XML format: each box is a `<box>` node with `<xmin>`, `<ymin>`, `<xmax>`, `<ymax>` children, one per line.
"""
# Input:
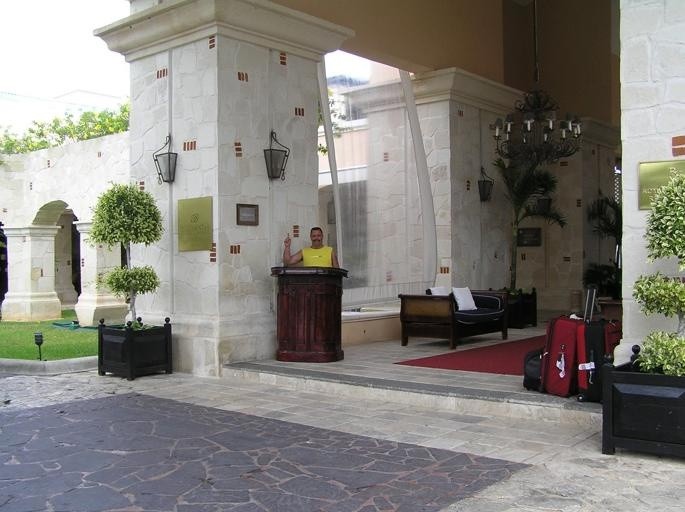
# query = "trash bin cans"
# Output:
<box><xmin>571</xmin><ymin>290</ymin><xmax>582</xmax><ymax>313</ymax></box>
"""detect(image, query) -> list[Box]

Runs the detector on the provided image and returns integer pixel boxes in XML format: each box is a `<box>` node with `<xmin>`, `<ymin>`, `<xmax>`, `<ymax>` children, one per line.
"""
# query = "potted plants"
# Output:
<box><xmin>583</xmin><ymin>197</ymin><xmax>622</xmax><ymax>321</ymax></box>
<box><xmin>88</xmin><ymin>184</ymin><xmax>172</xmax><ymax>380</ymax></box>
<box><xmin>601</xmin><ymin>167</ymin><xmax>685</xmax><ymax>457</ymax></box>
<box><xmin>491</xmin><ymin>158</ymin><xmax>567</xmax><ymax>328</ymax></box>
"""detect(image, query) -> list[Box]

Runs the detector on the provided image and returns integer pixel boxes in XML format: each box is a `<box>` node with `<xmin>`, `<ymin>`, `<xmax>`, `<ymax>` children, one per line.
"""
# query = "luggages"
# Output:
<box><xmin>539</xmin><ymin>283</ymin><xmax>598</xmax><ymax>400</ymax></box>
<box><xmin>575</xmin><ymin>317</ymin><xmax>622</xmax><ymax>403</ymax></box>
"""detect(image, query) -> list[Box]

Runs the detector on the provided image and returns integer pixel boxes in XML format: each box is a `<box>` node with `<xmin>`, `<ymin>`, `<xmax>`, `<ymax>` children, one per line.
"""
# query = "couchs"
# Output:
<box><xmin>398</xmin><ymin>293</ymin><xmax>507</xmax><ymax>350</ymax></box>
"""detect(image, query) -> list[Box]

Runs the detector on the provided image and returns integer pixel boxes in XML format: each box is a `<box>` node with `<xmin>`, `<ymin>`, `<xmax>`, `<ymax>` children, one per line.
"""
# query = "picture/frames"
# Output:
<box><xmin>517</xmin><ymin>228</ymin><xmax>541</xmax><ymax>246</ymax></box>
<box><xmin>236</xmin><ymin>204</ymin><xmax>259</xmax><ymax>226</ymax></box>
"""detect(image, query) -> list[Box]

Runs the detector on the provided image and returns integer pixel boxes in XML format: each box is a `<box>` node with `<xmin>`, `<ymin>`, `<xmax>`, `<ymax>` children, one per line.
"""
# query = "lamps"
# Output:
<box><xmin>489</xmin><ymin>0</ymin><xmax>583</xmax><ymax>162</ymax></box>
<box><xmin>478</xmin><ymin>167</ymin><xmax>495</xmax><ymax>201</ymax></box>
<box><xmin>264</xmin><ymin>131</ymin><xmax>290</xmax><ymax>181</ymax></box>
<box><xmin>153</xmin><ymin>136</ymin><xmax>177</xmax><ymax>183</ymax></box>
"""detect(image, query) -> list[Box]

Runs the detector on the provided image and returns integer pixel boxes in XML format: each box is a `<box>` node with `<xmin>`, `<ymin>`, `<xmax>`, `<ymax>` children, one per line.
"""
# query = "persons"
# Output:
<box><xmin>280</xmin><ymin>226</ymin><xmax>340</xmax><ymax>269</ymax></box>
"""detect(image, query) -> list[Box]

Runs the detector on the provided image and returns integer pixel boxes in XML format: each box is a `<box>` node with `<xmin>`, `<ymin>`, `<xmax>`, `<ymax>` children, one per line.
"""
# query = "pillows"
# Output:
<box><xmin>452</xmin><ymin>287</ymin><xmax>478</xmax><ymax>311</ymax></box>
<box><xmin>430</xmin><ymin>287</ymin><xmax>450</xmax><ymax>296</ymax></box>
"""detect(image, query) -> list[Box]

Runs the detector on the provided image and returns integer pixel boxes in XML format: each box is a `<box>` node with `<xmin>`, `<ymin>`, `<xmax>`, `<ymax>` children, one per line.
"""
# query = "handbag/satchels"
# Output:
<box><xmin>522</xmin><ymin>345</ymin><xmax>545</xmax><ymax>393</ymax></box>
